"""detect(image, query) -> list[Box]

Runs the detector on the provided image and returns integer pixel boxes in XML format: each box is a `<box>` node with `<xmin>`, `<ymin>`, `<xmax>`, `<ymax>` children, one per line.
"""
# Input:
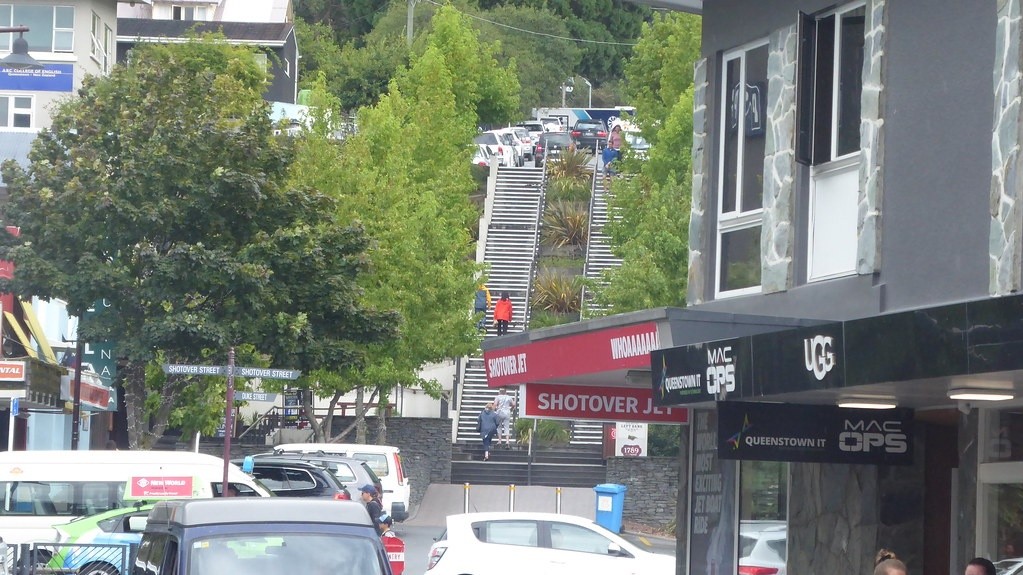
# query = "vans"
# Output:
<box><xmin>0</xmin><ymin>450</ymin><xmax>273</xmax><ymax>575</ymax></box>
<box><xmin>128</xmin><ymin>495</ymin><xmax>394</xmax><ymax>575</ymax></box>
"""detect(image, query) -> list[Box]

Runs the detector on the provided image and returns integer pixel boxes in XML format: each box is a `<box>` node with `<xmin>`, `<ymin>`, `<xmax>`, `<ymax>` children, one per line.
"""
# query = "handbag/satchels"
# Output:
<box><xmin>476</xmin><ymin>413</ymin><xmax>482</xmax><ymax>432</ymax></box>
<box><xmin>493</xmin><ymin>319</ymin><xmax>498</xmax><ymax>329</ymax></box>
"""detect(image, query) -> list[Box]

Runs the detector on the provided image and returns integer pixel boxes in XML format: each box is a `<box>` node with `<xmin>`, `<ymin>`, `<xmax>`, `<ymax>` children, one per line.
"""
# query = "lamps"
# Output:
<box><xmin>625</xmin><ymin>369</ymin><xmax>653</xmax><ymax>386</ymax></box>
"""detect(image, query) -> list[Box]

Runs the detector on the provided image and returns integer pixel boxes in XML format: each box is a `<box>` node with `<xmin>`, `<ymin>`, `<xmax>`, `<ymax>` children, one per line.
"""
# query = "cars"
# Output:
<box><xmin>993</xmin><ymin>557</ymin><xmax>1023</xmax><ymax>575</ymax></box>
<box><xmin>738</xmin><ymin>519</ymin><xmax>787</xmax><ymax>575</ymax></box>
<box><xmin>465</xmin><ymin>117</ymin><xmax>562</xmax><ymax>172</ymax></box>
<box><xmin>46</xmin><ymin>471</ymin><xmax>286</xmax><ymax>575</ymax></box>
<box><xmin>570</xmin><ymin>119</ymin><xmax>609</xmax><ymax>154</ymax></box>
<box><xmin>623</xmin><ymin>132</ymin><xmax>652</xmax><ymax>163</ymax></box>
<box><xmin>534</xmin><ymin>131</ymin><xmax>579</xmax><ymax>166</ymax></box>
<box><xmin>420</xmin><ymin>510</ymin><xmax>679</xmax><ymax>575</ymax></box>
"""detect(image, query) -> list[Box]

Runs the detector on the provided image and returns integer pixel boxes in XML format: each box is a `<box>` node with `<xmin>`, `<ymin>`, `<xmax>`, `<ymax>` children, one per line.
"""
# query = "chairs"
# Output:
<box><xmin>33</xmin><ymin>481</ymin><xmax>60</xmax><ymax>516</ymax></box>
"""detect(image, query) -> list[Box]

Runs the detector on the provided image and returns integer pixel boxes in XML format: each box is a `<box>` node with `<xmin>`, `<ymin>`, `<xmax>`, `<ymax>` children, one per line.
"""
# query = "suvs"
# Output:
<box><xmin>229</xmin><ymin>441</ymin><xmax>414</xmax><ymax>533</ymax></box>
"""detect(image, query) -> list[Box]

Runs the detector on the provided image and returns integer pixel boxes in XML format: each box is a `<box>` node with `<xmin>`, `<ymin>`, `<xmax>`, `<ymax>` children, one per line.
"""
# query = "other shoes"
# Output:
<box><xmin>484</xmin><ymin>457</ymin><xmax>488</xmax><ymax>461</ymax></box>
<box><xmin>496</xmin><ymin>444</ymin><xmax>502</xmax><ymax>449</ymax></box>
<box><xmin>506</xmin><ymin>444</ymin><xmax>510</xmax><ymax>449</ymax></box>
<box><xmin>488</xmin><ymin>452</ymin><xmax>491</xmax><ymax>457</ymax></box>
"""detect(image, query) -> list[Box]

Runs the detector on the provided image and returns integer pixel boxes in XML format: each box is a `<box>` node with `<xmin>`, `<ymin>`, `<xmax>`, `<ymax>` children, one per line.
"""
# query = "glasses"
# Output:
<box><xmin>362</xmin><ymin>492</ymin><xmax>368</xmax><ymax>495</ymax></box>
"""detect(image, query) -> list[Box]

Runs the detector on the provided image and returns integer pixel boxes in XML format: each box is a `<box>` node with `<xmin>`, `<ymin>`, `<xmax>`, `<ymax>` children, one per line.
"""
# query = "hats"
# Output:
<box><xmin>358</xmin><ymin>484</ymin><xmax>375</xmax><ymax>496</ymax></box>
<box><xmin>377</xmin><ymin>515</ymin><xmax>392</xmax><ymax>524</ymax></box>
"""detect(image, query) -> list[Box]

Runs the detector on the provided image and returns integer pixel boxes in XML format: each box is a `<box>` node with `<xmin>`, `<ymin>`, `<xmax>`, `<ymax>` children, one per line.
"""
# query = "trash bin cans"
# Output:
<box><xmin>593</xmin><ymin>484</ymin><xmax>627</xmax><ymax>534</ymax></box>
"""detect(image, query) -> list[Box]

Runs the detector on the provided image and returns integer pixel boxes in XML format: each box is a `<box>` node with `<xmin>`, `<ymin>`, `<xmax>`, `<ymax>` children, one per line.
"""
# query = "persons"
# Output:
<box><xmin>493</xmin><ymin>387</ymin><xmax>515</xmax><ymax>450</ymax></box>
<box><xmin>602</xmin><ymin>140</ymin><xmax>619</xmax><ymax>176</ymax></box>
<box><xmin>873</xmin><ymin>549</ymin><xmax>908</xmax><ymax>575</ymax></box>
<box><xmin>965</xmin><ymin>558</ymin><xmax>996</xmax><ymax>575</ymax></box>
<box><xmin>494</xmin><ymin>291</ymin><xmax>512</xmax><ymax>336</ymax></box>
<box><xmin>375</xmin><ymin>514</ymin><xmax>396</xmax><ymax>539</ymax></box>
<box><xmin>476</xmin><ymin>403</ymin><xmax>500</xmax><ymax>461</ymax></box>
<box><xmin>357</xmin><ymin>484</ymin><xmax>383</xmax><ymax>523</ymax></box>
<box><xmin>610</xmin><ymin>125</ymin><xmax>625</xmax><ymax>161</ymax></box>
<box><xmin>1004</xmin><ymin>544</ymin><xmax>1016</xmax><ymax>557</ymax></box>
<box><xmin>474</xmin><ymin>284</ymin><xmax>491</xmax><ymax>332</ymax></box>
